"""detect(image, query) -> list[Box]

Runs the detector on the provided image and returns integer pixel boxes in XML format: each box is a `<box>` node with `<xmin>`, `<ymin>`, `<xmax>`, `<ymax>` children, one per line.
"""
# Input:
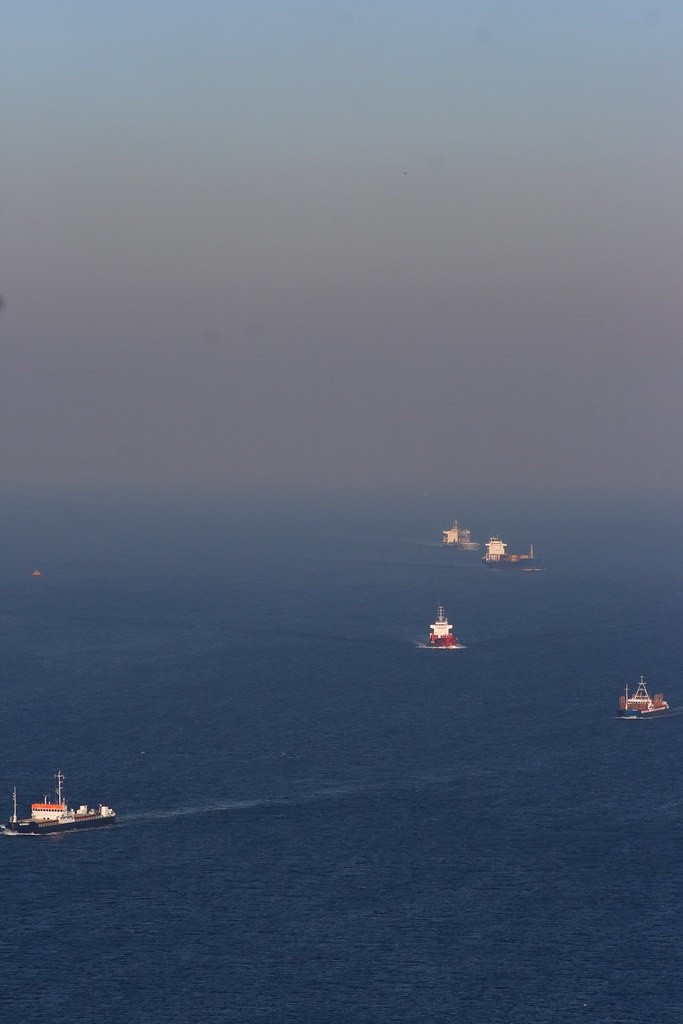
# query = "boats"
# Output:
<box><xmin>481</xmin><ymin>537</ymin><xmax>536</xmax><ymax>564</ymax></box>
<box><xmin>32</xmin><ymin>569</ymin><xmax>41</xmax><ymax>576</ymax></box>
<box><xmin>441</xmin><ymin>519</ymin><xmax>480</xmax><ymax>552</ymax></box>
<box><xmin>5</xmin><ymin>770</ymin><xmax>116</xmax><ymax>835</ymax></box>
<box><xmin>616</xmin><ymin>674</ymin><xmax>669</xmax><ymax>716</ymax></box>
<box><xmin>430</xmin><ymin>607</ymin><xmax>459</xmax><ymax>648</ymax></box>
